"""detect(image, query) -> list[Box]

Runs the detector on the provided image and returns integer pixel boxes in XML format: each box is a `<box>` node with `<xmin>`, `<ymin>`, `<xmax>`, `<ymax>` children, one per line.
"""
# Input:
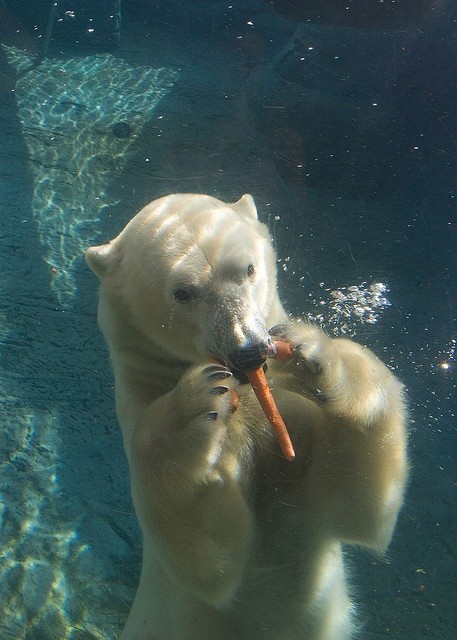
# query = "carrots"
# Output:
<box><xmin>247</xmin><ymin>367</ymin><xmax>295</xmax><ymax>462</ymax></box>
<box><xmin>269</xmin><ymin>339</ymin><xmax>293</xmax><ymax>361</ymax></box>
<box><xmin>207</xmin><ymin>354</ymin><xmax>238</xmax><ymax>414</ymax></box>
